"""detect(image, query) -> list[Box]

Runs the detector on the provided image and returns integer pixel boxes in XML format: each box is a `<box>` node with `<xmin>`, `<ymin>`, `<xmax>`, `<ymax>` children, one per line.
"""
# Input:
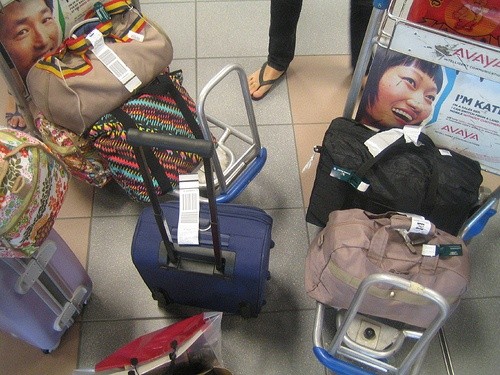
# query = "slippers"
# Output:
<box><xmin>248</xmin><ymin>62</ymin><xmax>287</xmax><ymax>101</ymax></box>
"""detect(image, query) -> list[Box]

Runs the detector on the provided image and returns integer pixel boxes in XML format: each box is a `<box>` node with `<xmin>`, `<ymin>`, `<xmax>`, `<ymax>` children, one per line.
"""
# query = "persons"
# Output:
<box><xmin>1</xmin><ymin>0</ymin><xmax>59</xmax><ymax>72</ymax></box>
<box><xmin>244</xmin><ymin>0</ymin><xmax>374</xmax><ymax>101</ymax></box>
<box><xmin>354</xmin><ymin>44</ymin><xmax>445</xmax><ymax>134</ymax></box>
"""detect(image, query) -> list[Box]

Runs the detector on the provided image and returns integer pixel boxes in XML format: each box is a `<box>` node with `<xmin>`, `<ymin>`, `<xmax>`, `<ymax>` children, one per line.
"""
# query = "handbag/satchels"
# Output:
<box><xmin>33</xmin><ymin>112</ymin><xmax>113</xmax><ymax>189</ymax></box>
<box><xmin>88</xmin><ymin>68</ymin><xmax>218</xmax><ymax>206</ymax></box>
<box><xmin>305</xmin><ymin>117</ymin><xmax>482</xmax><ymax>236</ymax></box>
<box><xmin>95</xmin><ymin>310</ymin><xmax>223</xmax><ymax>374</ymax></box>
<box><xmin>26</xmin><ymin>1</ymin><xmax>173</xmax><ymax>139</ymax></box>
<box><xmin>0</xmin><ymin>126</ymin><xmax>71</xmax><ymax>259</ymax></box>
<box><xmin>305</xmin><ymin>207</ymin><xmax>470</xmax><ymax>329</ymax></box>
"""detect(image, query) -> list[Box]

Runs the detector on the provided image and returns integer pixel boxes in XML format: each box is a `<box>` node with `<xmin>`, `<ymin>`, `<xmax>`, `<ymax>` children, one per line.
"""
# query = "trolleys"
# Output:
<box><xmin>0</xmin><ymin>0</ymin><xmax>266</xmax><ymax>203</ymax></box>
<box><xmin>310</xmin><ymin>1</ymin><xmax>500</xmax><ymax>371</ymax></box>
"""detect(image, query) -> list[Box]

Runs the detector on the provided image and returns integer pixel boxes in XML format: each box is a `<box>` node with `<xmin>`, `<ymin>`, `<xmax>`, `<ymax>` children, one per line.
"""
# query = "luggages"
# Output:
<box><xmin>126</xmin><ymin>128</ymin><xmax>274</xmax><ymax>319</ymax></box>
<box><xmin>1</xmin><ymin>227</ymin><xmax>93</xmax><ymax>354</ymax></box>
